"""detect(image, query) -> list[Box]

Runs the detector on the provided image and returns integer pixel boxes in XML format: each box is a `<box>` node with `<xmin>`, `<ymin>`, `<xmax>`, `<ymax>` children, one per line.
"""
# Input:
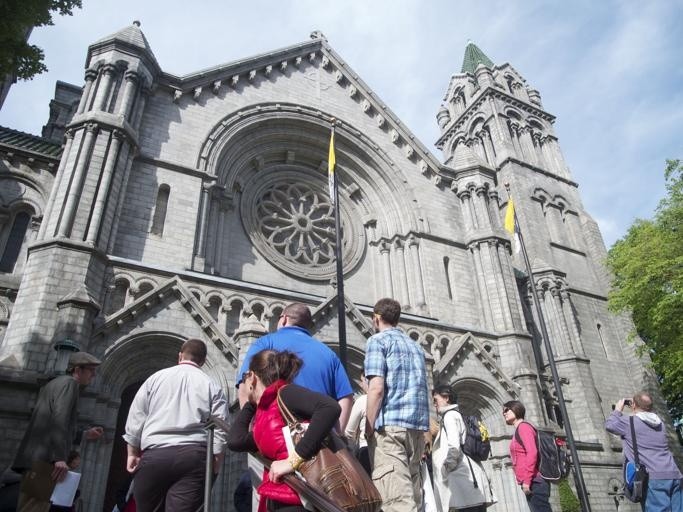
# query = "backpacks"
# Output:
<box><xmin>442</xmin><ymin>409</ymin><xmax>490</xmax><ymax>461</ymax></box>
<box><xmin>515</xmin><ymin>422</ymin><xmax>568</xmax><ymax>480</ymax></box>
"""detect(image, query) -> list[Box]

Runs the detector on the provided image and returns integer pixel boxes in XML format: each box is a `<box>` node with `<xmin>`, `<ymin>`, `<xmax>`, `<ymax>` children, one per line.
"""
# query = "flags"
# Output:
<box><xmin>327</xmin><ymin>128</ymin><xmax>335</xmax><ymax>208</ymax></box>
<box><xmin>503</xmin><ymin>194</ymin><xmax>524</xmax><ymax>255</ymax></box>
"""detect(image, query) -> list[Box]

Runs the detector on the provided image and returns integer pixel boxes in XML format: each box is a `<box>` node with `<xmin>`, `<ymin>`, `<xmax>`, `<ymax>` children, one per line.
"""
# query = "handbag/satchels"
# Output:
<box><xmin>290</xmin><ymin>424</ymin><xmax>382</xmax><ymax>511</ymax></box>
<box><xmin>622</xmin><ymin>459</ymin><xmax>646</xmax><ymax>503</ymax></box>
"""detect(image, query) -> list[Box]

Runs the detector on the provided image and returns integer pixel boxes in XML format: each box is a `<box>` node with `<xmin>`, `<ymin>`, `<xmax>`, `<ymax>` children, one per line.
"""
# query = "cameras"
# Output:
<box><xmin>624</xmin><ymin>400</ymin><xmax>632</xmax><ymax>405</ymax></box>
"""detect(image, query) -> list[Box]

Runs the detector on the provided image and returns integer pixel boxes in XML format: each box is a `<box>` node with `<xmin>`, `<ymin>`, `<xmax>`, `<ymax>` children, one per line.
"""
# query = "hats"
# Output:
<box><xmin>69</xmin><ymin>352</ymin><xmax>101</xmax><ymax>367</ymax></box>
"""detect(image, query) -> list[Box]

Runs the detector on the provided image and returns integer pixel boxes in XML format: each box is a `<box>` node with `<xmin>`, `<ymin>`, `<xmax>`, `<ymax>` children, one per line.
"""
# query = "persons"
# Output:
<box><xmin>501</xmin><ymin>399</ymin><xmax>553</xmax><ymax>511</ymax></box>
<box><xmin>604</xmin><ymin>393</ymin><xmax>682</xmax><ymax>511</ymax></box>
<box><xmin>361</xmin><ymin>298</ymin><xmax>429</xmax><ymax>511</ymax></box>
<box><xmin>344</xmin><ymin>367</ymin><xmax>373</xmax><ymax>478</ymax></box>
<box><xmin>121</xmin><ymin>338</ymin><xmax>231</xmax><ymax>510</ymax></box>
<box><xmin>227</xmin><ymin>302</ymin><xmax>352</xmax><ymax>511</ymax></box>
<box><xmin>431</xmin><ymin>386</ymin><xmax>497</xmax><ymax>512</ymax></box>
<box><xmin>10</xmin><ymin>350</ymin><xmax>103</xmax><ymax>512</ymax></box>
<box><xmin>54</xmin><ymin>451</ymin><xmax>81</xmax><ymax>508</ymax></box>
